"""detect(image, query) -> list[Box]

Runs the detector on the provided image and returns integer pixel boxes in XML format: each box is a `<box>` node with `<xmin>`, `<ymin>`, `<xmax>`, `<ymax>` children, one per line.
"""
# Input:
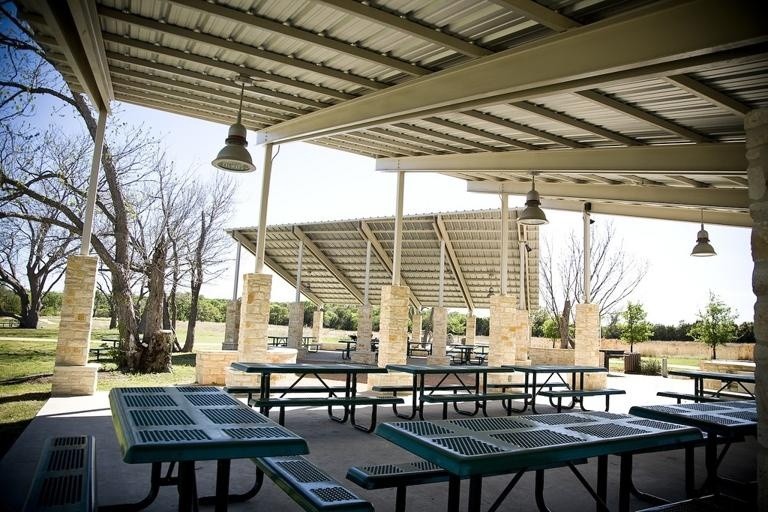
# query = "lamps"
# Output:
<box><xmin>208</xmin><ymin>72</ymin><xmax>260</xmax><ymax>178</ymax></box>
<box><xmin>688</xmin><ymin>206</ymin><xmax>719</xmax><ymax>258</ymax></box>
<box><xmin>514</xmin><ymin>170</ymin><xmax>550</xmax><ymax>230</ymax></box>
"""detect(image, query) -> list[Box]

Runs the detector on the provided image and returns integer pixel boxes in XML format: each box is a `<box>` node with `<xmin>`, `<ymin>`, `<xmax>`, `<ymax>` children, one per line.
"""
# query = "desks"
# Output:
<box><xmin>385</xmin><ymin>364</ymin><xmax>515</xmax><ymax>420</ymax></box>
<box><xmin>626</xmin><ymin>400</ymin><xmax>760</xmax><ymax>511</ymax></box>
<box><xmin>668</xmin><ymin>370</ymin><xmax>756</xmax><ymax>404</ymax></box>
<box><xmin>502</xmin><ymin>364</ymin><xmax>609</xmax><ymax>411</ymax></box>
<box><xmin>375</xmin><ymin>410</ymin><xmax>703</xmax><ymax>510</ymax></box>
<box><xmin>106</xmin><ymin>382</ymin><xmax>311</xmax><ymax>510</ymax></box>
<box><xmin>229</xmin><ymin>360</ymin><xmax>389</xmax><ymax>423</ymax></box>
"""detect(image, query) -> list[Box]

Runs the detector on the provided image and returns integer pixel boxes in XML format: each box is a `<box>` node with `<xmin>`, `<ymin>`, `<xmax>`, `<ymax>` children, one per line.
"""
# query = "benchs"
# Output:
<box><xmin>485</xmin><ymin>382</ymin><xmax>572</xmax><ymax>411</ymax></box>
<box><xmin>370</xmin><ymin>384</ymin><xmax>476</xmax><ymax>418</ymax></box>
<box><xmin>223</xmin><ymin>384</ymin><xmax>357</xmax><ymax>423</ymax></box>
<box><xmin>697</xmin><ymin>389</ymin><xmax>757</xmax><ymax>402</ymax></box>
<box><xmin>18</xmin><ymin>431</ymin><xmax>100</xmax><ymax>510</ymax></box>
<box><xmin>656</xmin><ymin>388</ymin><xmax>724</xmax><ymax>404</ymax></box>
<box><xmin>343</xmin><ymin>456</ymin><xmax>611</xmax><ymax>512</ymax></box>
<box><xmin>416</xmin><ymin>392</ymin><xmax>533</xmax><ymax>418</ymax></box>
<box><xmin>535</xmin><ymin>387</ymin><xmax>627</xmax><ymax>412</ymax></box>
<box><xmin>248</xmin><ymin>452</ymin><xmax>377</xmax><ymax>511</ymax></box>
<box><xmin>90</xmin><ymin>337</ymin><xmax>530</xmax><ymax>365</ymax></box>
<box><xmin>247</xmin><ymin>395</ymin><xmax>405</xmax><ymax>435</ymax></box>
